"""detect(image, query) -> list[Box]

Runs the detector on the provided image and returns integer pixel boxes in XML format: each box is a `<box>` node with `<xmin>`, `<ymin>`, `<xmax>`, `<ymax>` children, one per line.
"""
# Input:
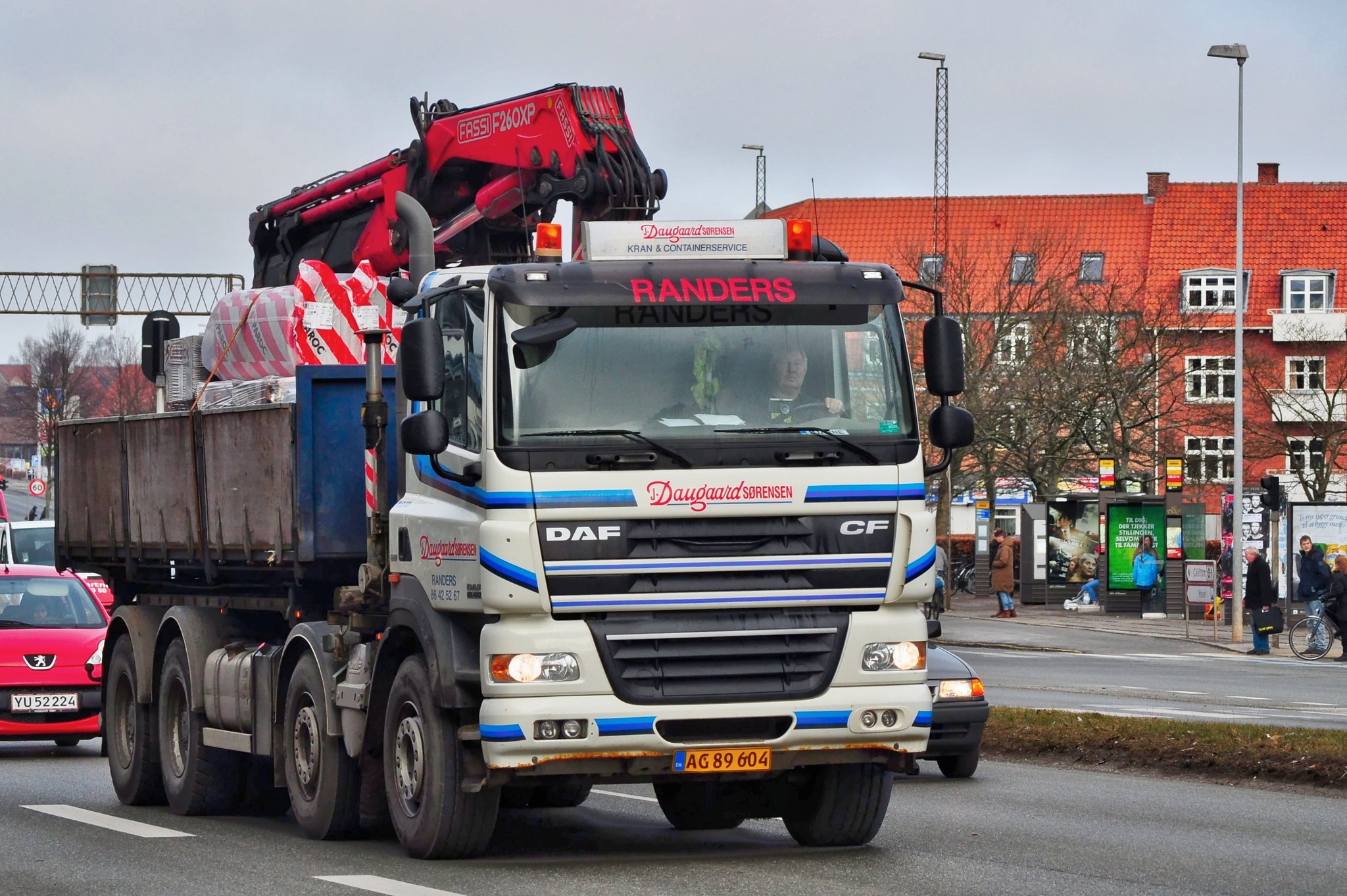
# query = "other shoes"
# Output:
<box><xmin>990</xmin><ymin>609</ymin><xmax>1003</xmax><ymax>617</ymax></box>
<box><xmin>996</xmin><ymin>609</ymin><xmax>1011</xmax><ymax>618</ymax></box>
<box><xmin>1246</xmin><ymin>648</ymin><xmax>1270</xmax><ymax>656</ymax></box>
<box><xmin>1334</xmin><ymin>652</ymin><xmax>1347</xmax><ymax>662</ymax></box>
<box><xmin>1298</xmin><ymin>649</ymin><xmax>1312</xmax><ymax>655</ymax></box>
<box><xmin>939</xmin><ymin>604</ymin><xmax>945</xmax><ymax>614</ymax></box>
<box><xmin>1009</xmin><ymin>608</ymin><xmax>1018</xmax><ymax>618</ymax></box>
<box><xmin>1310</xmin><ymin>649</ymin><xmax>1325</xmax><ymax>656</ymax></box>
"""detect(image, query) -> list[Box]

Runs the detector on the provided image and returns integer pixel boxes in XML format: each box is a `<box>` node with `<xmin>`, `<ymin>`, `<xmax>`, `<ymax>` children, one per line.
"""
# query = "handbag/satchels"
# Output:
<box><xmin>1252</xmin><ymin>605</ymin><xmax>1284</xmax><ymax>637</ymax></box>
<box><xmin>1064</xmin><ymin>588</ymin><xmax>1086</xmax><ymax>611</ymax></box>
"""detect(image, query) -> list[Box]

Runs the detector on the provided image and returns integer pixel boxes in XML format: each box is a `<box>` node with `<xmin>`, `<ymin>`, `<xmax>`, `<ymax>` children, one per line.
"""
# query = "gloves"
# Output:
<box><xmin>1320</xmin><ymin>594</ymin><xmax>1326</xmax><ymax>602</ymax></box>
<box><xmin>1316</xmin><ymin>590</ymin><xmax>1324</xmax><ymax>596</ymax></box>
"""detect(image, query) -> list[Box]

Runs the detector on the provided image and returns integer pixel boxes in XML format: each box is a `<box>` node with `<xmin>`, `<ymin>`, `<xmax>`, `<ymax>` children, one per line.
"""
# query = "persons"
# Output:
<box><xmin>1297</xmin><ymin>535</ymin><xmax>1332</xmax><ymax>656</ymax></box>
<box><xmin>23</xmin><ymin>600</ymin><xmax>75</xmax><ymax>625</ymax></box>
<box><xmin>1317</xmin><ymin>554</ymin><xmax>1347</xmax><ymax>661</ymax></box>
<box><xmin>1132</xmin><ymin>536</ymin><xmax>1161</xmax><ymax>579</ymax></box>
<box><xmin>1069</xmin><ymin>553</ymin><xmax>1100</xmax><ymax>582</ymax></box>
<box><xmin>990</xmin><ymin>525</ymin><xmax>1019</xmax><ymax>619</ymax></box>
<box><xmin>935</xmin><ymin>543</ymin><xmax>948</xmax><ymax>614</ymax></box>
<box><xmin>25</xmin><ymin>503</ymin><xmax>47</xmax><ymax>521</ymax></box>
<box><xmin>1242</xmin><ymin>546</ymin><xmax>1274</xmax><ymax>655</ymax></box>
<box><xmin>1081</xmin><ymin>545</ymin><xmax>1100</xmax><ymax>605</ymax></box>
<box><xmin>1242</xmin><ymin>522</ymin><xmax>1264</xmax><ymax>540</ymax></box>
<box><xmin>740</xmin><ymin>337</ymin><xmax>846</xmax><ymax>427</ymax></box>
<box><xmin>1242</xmin><ymin>495</ymin><xmax>1264</xmax><ymax>514</ymax></box>
<box><xmin>1051</xmin><ymin>512</ymin><xmax>1078</xmax><ymax>539</ymax></box>
<box><xmin>1133</xmin><ymin>544</ymin><xmax>1159</xmax><ymax>619</ymax></box>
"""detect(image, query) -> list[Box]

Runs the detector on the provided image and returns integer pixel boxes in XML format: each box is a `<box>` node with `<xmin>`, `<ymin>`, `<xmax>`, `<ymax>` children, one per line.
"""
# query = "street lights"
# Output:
<box><xmin>918</xmin><ymin>51</ymin><xmax>950</xmax><ymax>318</ymax></box>
<box><xmin>742</xmin><ymin>143</ymin><xmax>768</xmax><ymax>218</ymax></box>
<box><xmin>1207</xmin><ymin>43</ymin><xmax>1244</xmax><ymax>642</ymax></box>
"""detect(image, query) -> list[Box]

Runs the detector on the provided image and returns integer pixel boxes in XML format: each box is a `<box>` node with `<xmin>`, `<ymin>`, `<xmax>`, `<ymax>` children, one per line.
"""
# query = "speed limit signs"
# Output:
<box><xmin>29</xmin><ymin>479</ymin><xmax>46</xmax><ymax>495</ymax></box>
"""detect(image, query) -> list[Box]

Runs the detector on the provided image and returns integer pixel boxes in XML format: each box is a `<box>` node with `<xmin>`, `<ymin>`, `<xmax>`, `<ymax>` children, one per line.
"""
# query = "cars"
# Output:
<box><xmin>914</xmin><ymin>642</ymin><xmax>989</xmax><ymax>779</ymax></box>
<box><xmin>0</xmin><ymin>489</ymin><xmax>115</xmax><ymax>746</ymax></box>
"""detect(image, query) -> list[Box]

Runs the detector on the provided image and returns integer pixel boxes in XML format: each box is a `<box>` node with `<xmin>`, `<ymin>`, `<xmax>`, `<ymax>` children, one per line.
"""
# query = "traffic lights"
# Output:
<box><xmin>1260</xmin><ymin>476</ymin><xmax>1280</xmax><ymax>510</ymax></box>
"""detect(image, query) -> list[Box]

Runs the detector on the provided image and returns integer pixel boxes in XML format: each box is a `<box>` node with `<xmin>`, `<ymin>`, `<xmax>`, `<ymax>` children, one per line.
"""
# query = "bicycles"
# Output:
<box><xmin>1289</xmin><ymin>596</ymin><xmax>1342</xmax><ymax>661</ymax></box>
<box><xmin>923</xmin><ymin>558</ymin><xmax>995</xmax><ymax>620</ymax></box>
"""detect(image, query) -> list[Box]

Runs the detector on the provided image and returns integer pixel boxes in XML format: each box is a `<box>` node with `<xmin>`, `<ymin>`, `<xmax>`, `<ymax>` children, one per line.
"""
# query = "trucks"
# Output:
<box><xmin>53</xmin><ymin>220</ymin><xmax>973</xmax><ymax>861</ymax></box>
